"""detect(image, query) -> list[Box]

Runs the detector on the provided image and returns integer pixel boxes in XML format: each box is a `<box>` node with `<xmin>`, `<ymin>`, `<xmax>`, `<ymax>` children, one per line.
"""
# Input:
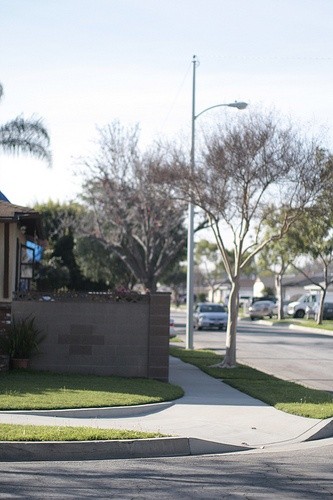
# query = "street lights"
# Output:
<box><xmin>186</xmin><ymin>53</ymin><xmax>249</xmax><ymax>354</ymax></box>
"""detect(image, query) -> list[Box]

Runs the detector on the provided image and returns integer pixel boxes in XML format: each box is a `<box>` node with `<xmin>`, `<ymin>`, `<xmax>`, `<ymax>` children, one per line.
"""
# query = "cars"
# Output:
<box><xmin>192</xmin><ymin>302</ymin><xmax>228</xmax><ymax>332</ymax></box>
<box><xmin>312</xmin><ymin>300</ymin><xmax>333</xmax><ymax>320</ymax></box>
<box><xmin>249</xmin><ymin>300</ymin><xmax>274</xmax><ymax>321</ymax></box>
<box><xmin>272</xmin><ymin>301</ymin><xmax>293</xmax><ymax>318</ymax></box>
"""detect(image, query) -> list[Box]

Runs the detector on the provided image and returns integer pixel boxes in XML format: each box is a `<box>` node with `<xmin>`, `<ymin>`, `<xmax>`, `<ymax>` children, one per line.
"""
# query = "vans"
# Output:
<box><xmin>287</xmin><ymin>293</ymin><xmax>333</xmax><ymax>318</ymax></box>
